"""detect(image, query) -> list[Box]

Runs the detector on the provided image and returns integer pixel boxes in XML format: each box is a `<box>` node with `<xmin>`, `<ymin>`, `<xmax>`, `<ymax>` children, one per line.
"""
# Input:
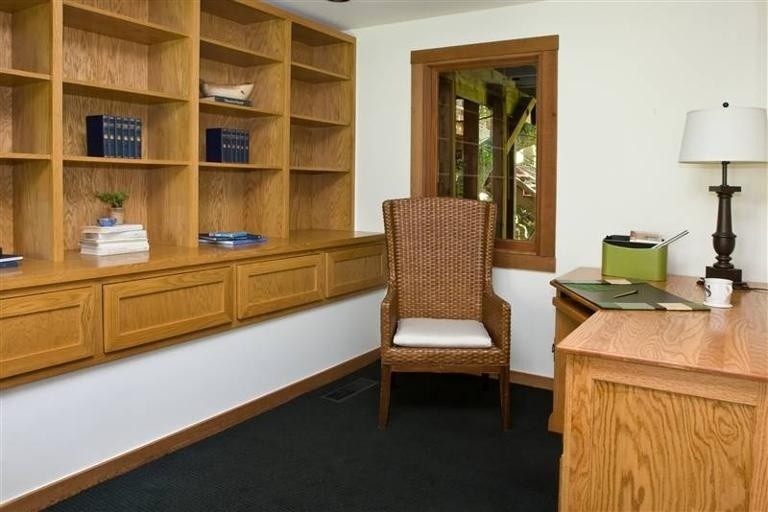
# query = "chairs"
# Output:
<box><xmin>380</xmin><ymin>197</ymin><xmax>511</xmax><ymax>428</ymax></box>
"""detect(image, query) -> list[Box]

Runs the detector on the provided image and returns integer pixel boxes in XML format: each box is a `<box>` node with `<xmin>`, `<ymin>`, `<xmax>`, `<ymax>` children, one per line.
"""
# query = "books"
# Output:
<box><xmin>0</xmin><ymin>253</ymin><xmax>23</xmax><ymax>268</ymax></box>
<box><xmin>199</xmin><ymin>231</ymin><xmax>265</xmax><ymax>246</ymax></box>
<box><xmin>86</xmin><ymin>111</ymin><xmax>141</xmax><ymax>158</ymax></box>
<box><xmin>206</xmin><ymin>128</ymin><xmax>249</xmax><ymax>163</ymax></box>
<box><xmin>198</xmin><ymin>96</ymin><xmax>250</xmax><ymax>106</ymax></box>
<box><xmin>81</xmin><ymin>224</ymin><xmax>150</xmax><ymax>256</ymax></box>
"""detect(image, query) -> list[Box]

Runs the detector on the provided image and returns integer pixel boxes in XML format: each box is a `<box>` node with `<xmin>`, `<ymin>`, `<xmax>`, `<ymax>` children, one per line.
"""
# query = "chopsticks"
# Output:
<box><xmin>650</xmin><ymin>229</ymin><xmax>689</xmax><ymax>250</ymax></box>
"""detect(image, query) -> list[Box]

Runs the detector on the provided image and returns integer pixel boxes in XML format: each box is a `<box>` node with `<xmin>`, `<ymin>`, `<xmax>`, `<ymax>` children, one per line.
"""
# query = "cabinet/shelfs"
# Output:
<box><xmin>0</xmin><ymin>0</ymin><xmax>388</xmax><ymax>389</ymax></box>
<box><xmin>437</xmin><ymin>74</ymin><xmax>507</xmax><ymax>241</ymax></box>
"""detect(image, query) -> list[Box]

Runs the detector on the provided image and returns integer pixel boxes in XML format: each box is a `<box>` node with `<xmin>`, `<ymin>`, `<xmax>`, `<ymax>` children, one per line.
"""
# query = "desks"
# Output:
<box><xmin>549</xmin><ymin>267</ymin><xmax>768</xmax><ymax>512</ymax></box>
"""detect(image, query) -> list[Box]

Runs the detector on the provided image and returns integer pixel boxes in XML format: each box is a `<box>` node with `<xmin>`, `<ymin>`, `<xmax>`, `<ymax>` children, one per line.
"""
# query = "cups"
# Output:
<box><xmin>703</xmin><ymin>276</ymin><xmax>734</xmax><ymax>310</ymax></box>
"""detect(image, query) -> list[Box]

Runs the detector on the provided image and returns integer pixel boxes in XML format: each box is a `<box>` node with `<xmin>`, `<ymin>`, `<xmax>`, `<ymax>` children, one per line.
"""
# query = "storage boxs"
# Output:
<box><xmin>602</xmin><ymin>242</ymin><xmax>667</xmax><ymax>282</ymax></box>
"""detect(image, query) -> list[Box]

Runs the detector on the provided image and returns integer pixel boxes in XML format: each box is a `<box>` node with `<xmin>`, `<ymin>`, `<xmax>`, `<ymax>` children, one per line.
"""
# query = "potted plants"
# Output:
<box><xmin>97</xmin><ymin>192</ymin><xmax>129</xmax><ymax>225</ymax></box>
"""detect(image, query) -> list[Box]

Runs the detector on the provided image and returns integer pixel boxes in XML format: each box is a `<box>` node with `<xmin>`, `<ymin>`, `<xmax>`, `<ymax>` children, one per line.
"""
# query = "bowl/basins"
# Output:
<box><xmin>199</xmin><ymin>79</ymin><xmax>254</xmax><ymax>101</ymax></box>
<box><xmin>97</xmin><ymin>217</ymin><xmax>118</xmax><ymax>226</ymax></box>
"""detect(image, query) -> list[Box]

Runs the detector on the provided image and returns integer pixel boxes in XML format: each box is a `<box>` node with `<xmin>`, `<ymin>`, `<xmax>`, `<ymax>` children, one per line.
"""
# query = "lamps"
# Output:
<box><xmin>679</xmin><ymin>103</ymin><xmax>768</xmax><ymax>290</ymax></box>
